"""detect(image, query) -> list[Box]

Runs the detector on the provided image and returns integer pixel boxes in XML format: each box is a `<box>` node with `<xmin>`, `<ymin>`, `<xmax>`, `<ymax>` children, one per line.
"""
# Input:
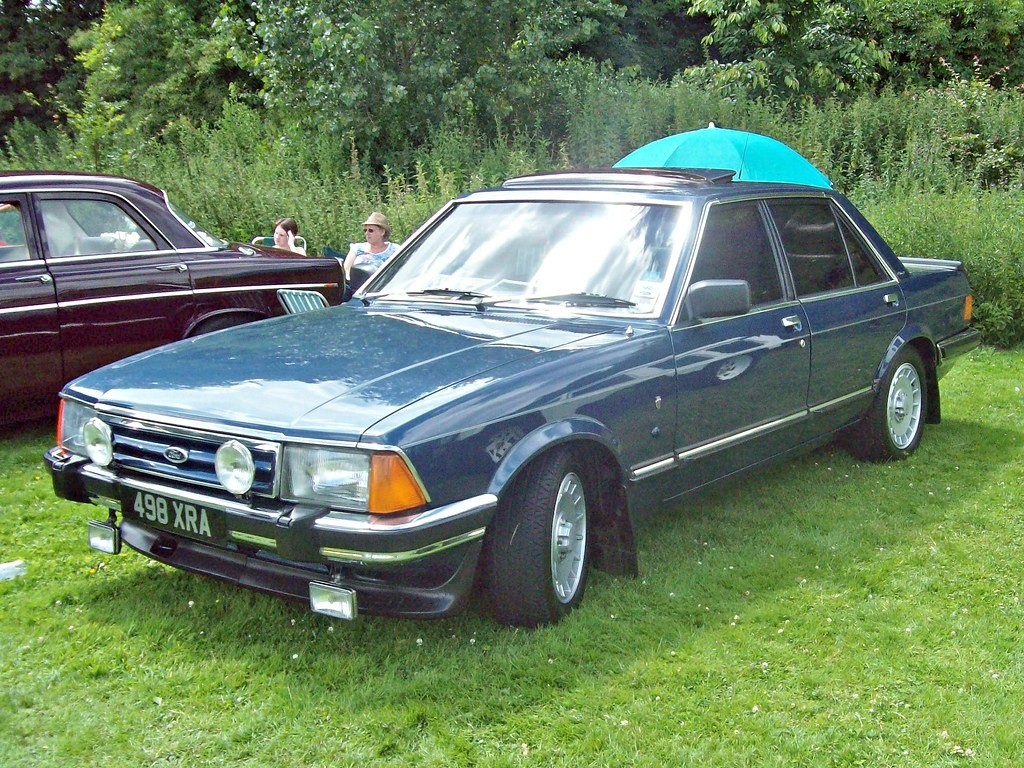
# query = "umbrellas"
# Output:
<box><xmin>611</xmin><ymin>121</ymin><xmax>834</xmax><ymax>190</ymax></box>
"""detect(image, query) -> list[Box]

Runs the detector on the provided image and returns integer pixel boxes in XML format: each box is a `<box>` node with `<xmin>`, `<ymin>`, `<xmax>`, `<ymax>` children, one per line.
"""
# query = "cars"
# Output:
<box><xmin>0</xmin><ymin>172</ymin><xmax>344</xmax><ymax>423</ymax></box>
<box><xmin>40</xmin><ymin>165</ymin><xmax>982</xmax><ymax>625</ymax></box>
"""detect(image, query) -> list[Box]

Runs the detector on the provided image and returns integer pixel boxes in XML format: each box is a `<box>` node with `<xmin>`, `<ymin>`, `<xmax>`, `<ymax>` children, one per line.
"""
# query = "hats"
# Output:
<box><xmin>362</xmin><ymin>212</ymin><xmax>391</xmax><ymax>236</ymax></box>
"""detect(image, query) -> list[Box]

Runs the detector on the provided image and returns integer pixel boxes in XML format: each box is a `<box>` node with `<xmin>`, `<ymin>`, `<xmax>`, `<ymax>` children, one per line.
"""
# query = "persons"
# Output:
<box><xmin>272</xmin><ymin>218</ymin><xmax>306</xmax><ymax>256</ymax></box>
<box><xmin>344</xmin><ymin>212</ymin><xmax>401</xmax><ymax>302</ymax></box>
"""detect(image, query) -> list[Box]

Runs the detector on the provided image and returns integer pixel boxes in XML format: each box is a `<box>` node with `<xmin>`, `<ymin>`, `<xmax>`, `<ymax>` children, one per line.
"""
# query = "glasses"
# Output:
<box><xmin>364</xmin><ymin>228</ymin><xmax>383</xmax><ymax>232</ymax></box>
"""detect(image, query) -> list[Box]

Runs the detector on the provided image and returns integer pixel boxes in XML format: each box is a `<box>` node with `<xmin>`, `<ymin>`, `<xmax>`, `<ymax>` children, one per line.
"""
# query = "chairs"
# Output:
<box><xmin>78</xmin><ymin>237</ymin><xmax>119</xmax><ymax>254</ymax></box>
<box><xmin>129</xmin><ymin>239</ymin><xmax>157</xmax><ymax>251</ymax></box>
<box><xmin>252</xmin><ymin>235</ymin><xmax>307</xmax><ymax>252</ymax></box>
<box><xmin>323</xmin><ymin>246</ymin><xmax>353</xmax><ymax>303</ymax></box>
